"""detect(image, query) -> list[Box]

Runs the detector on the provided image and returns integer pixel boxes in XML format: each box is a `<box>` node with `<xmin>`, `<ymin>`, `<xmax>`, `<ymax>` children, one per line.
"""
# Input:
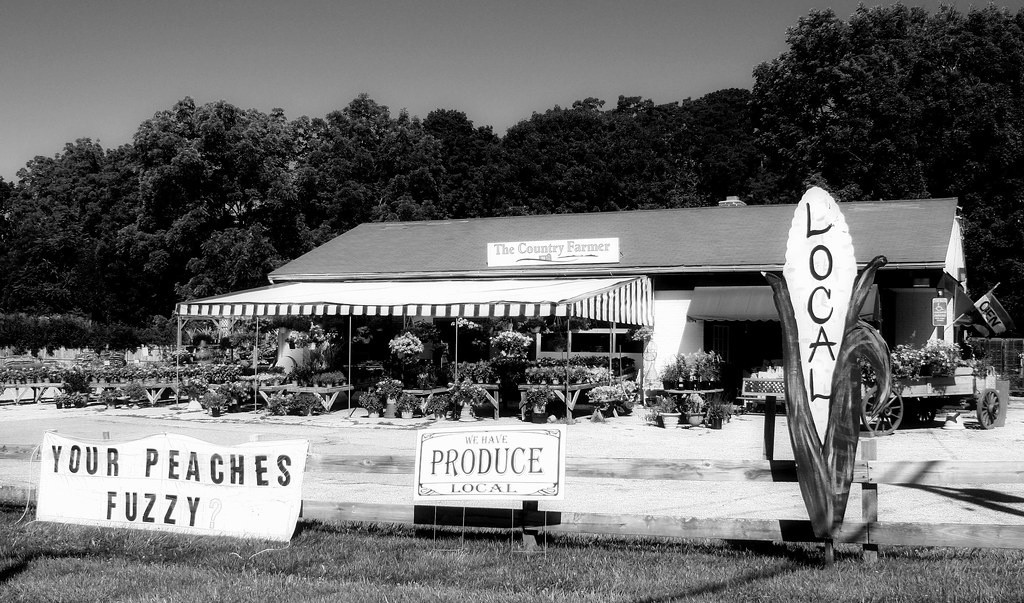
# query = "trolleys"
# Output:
<box><xmin>860</xmin><ymin>363</ymin><xmax>1004</xmax><ymax>437</ymax></box>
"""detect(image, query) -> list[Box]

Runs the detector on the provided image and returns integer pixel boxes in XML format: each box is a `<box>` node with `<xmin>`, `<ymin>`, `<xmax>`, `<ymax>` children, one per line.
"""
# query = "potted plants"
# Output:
<box><xmin>119</xmin><ymin>382</ymin><xmax>149</xmax><ymax>409</ymax></box>
<box><xmin>706</xmin><ymin>393</ymin><xmax>733</xmax><ymax>430</ymax></box>
<box><xmin>659</xmin><ymin>363</ymin><xmax>679</xmax><ymax>390</ymax></box>
<box><xmin>287</xmin><ymin>371</ymin><xmax>299</xmax><ymax>386</ymax></box>
<box><xmin>643</xmin><ymin>395</ymin><xmax>681</xmax><ymax>429</ymax></box>
<box><xmin>200</xmin><ymin>391</ymin><xmax>228</xmax><ymax>417</ymax></box>
<box><xmin>393</xmin><ymin>391</ymin><xmax>423</xmax><ymax>419</ymax></box>
<box><xmin>673</xmin><ymin>349</ymin><xmax>725</xmax><ymax>391</ymax></box>
<box><xmin>426</xmin><ymin>393</ymin><xmax>451</xmax><ymax>420</ymax></box>
<box><xmin>309</xmin><ymin>373</ymin><xmax>320</xmax><ymax>387</ymax></box>
<box><xmin>519</xmin><ymin>384</ymin><xmax>556</xmax><ymax>414</ymax></box>
<box><xmin>54</xmin><ymin>390</ymin><xmax>64</xmax><ymax>409</ymax></box>
<box><xmin>332</xmin><ymin>370</ymin><xmax>340</xmax><ymax>386</ymax></box>
<box><xmin>63</xmin><ymin>393</ymin><xmax>72</xmax><ymax>409</ymax></box>
<box><xmin>337</xmin><ymin>371</ymin><xmax>347</xmax><ymax>386</ymax></box>
<box><xmin>358</xmin><ymin>389</ymin><xmax>386</xmax><ymax>419</ymax></box>
<box><xmin>320</xmin><ymin>372</ymin><xmax>337</xmax><ymax>388</ymax></box>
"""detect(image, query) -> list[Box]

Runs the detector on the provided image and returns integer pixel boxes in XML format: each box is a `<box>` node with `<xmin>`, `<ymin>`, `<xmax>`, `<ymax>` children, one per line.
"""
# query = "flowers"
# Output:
<box><xmin>679</xmin><ymin>393</ymin><xmax>713</xmax><ymax>415</ymax></box>
<box><xmin>0</xmin><ymin>317</ymin><xmax>656</xmax><ymax>411</ymax></box>
<box><xmin>857</xmin><ymin>338</ymin><xmax>995</xmax><ymax>387</ymax></box>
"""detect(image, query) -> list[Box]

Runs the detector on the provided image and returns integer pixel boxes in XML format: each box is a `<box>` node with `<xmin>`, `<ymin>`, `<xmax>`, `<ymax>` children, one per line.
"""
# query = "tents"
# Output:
<box><xmin>175</xmin><ymin>276</ymin><xmax>655</xmax><ymax>418</ymax></box>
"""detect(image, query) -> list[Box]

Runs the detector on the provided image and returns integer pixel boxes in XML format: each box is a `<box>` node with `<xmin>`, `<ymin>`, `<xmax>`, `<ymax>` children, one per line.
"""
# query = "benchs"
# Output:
<box><xmin>736</xmin><ymin>377</ymin><xmax>785</xmax><ymax>407</ymax></box>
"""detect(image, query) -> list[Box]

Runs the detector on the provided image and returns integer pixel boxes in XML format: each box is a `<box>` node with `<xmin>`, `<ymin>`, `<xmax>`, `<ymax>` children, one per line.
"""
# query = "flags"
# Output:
<box><xmin>954</xmin><ymin>292</ymin><xmax>1016</xmax><ymax>340</ymax></box>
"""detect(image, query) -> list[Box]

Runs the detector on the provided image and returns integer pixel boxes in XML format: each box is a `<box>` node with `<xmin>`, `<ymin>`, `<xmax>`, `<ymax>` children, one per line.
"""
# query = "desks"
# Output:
<box><xmin>665</xmin><ymin>388</ymin><xmax>724</xmax><ymax>428</ymax></box>
<box><xmin>518</xmin><ymin>380</ymin><xmax>616</xmax><ymax>422</ymax></box>
<box><xmin>0</xmin><ymin>382</ymin><xmax>179</xmax><ymax>408</ymax></box>
<box><xmin>473</xmin><ymin>383</ymin><xmax>513</xmax><ymax>420</ymax></box>
<box><xmin>402</xmin><ymin>387</ymin><xmax>447</xmax><ymax>418</ymax></box>
<box><xmin>258</xmin><ymin>384</ymin><xmax>354</xmax><ymax>412</ymax></box>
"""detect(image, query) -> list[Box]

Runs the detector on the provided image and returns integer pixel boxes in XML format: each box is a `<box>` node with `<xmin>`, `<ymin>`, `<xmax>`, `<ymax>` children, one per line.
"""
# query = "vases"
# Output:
<box><xmin>685</xmin><ymin>412</ymin><xmax>706</xmax><ymax>426</ymax></box>
<box><xmin>80</xmin><ymin>392</ymin><xmax>88</xmax><ymax>405</ymax></box>
